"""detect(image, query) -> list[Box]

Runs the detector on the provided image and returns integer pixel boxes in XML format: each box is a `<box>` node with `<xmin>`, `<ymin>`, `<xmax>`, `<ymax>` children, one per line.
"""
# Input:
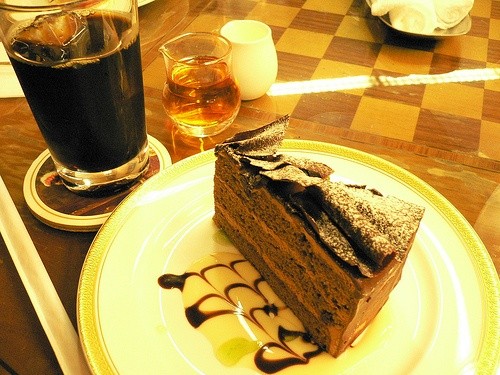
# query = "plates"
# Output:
<box><xmin>75</xmin><ymin>139</ymin><xmax>500</xmax><ymax>375</ymax></box>
<box><xmin>365</xmin><ymin>0</ymin><xmax>473</xmax><ymax>38</ymax></box>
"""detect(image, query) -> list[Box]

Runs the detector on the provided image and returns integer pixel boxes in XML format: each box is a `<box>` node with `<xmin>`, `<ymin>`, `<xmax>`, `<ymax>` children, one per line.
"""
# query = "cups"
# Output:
<box><xmin>0</xmin><ymin>0</ymin><xmax>150</xmax><ymax>197</ymax></box>
<box><xmin>214</xmin><ymin>19</ymin><xmax>278</xmax><ymax>100</ymax></box>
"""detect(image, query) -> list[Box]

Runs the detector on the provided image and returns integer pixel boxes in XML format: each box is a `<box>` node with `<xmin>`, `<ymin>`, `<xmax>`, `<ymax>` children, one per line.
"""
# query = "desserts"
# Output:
<box><xmin>213</xmin><ymin>114</ymin><xmax>425</xmax><ymax>358</ymax></box>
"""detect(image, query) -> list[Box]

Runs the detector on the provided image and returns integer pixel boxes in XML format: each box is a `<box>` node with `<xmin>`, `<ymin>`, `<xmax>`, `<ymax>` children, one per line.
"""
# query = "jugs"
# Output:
<box><xmin>157</xmin><ymin>32</ymin><xmax>241</xmax><ymax>137</ymax></box>
